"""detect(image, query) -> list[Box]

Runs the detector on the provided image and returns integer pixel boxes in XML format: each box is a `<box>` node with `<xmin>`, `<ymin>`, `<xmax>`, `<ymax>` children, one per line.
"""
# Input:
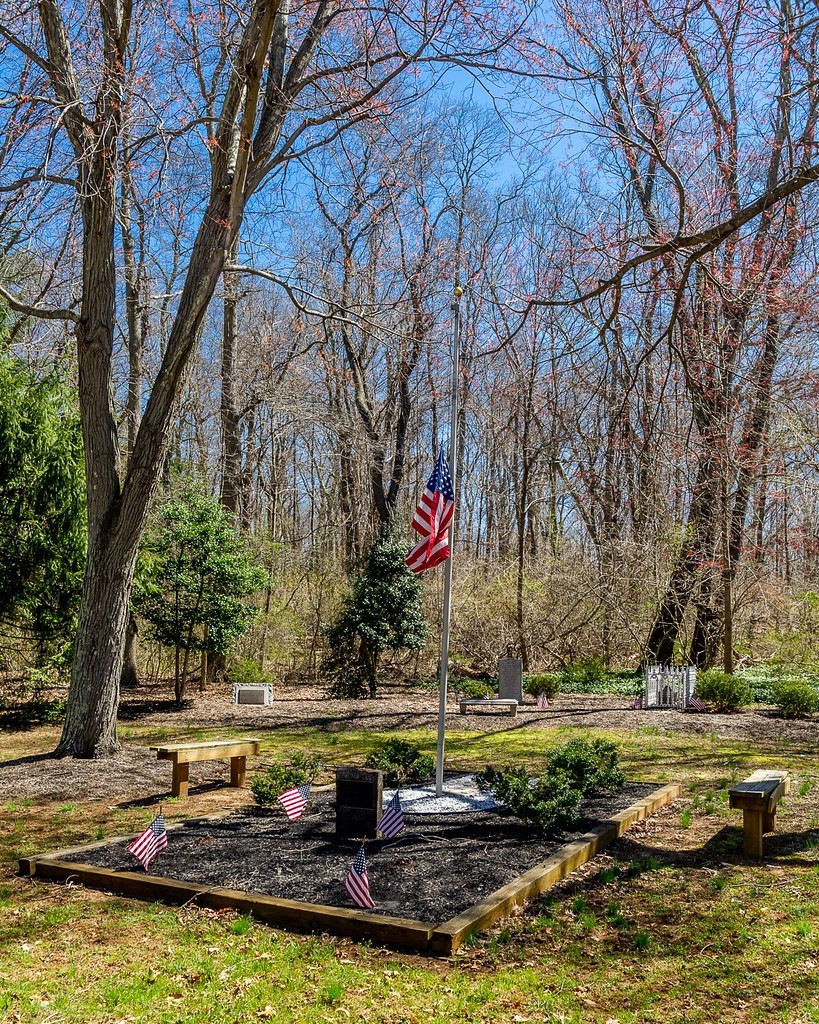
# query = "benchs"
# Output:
<box><xmin>728</xmin><ymin>769</ymin><xmax>790</xmax><ymax>859</ymax></box>
<box><xmin>460</xmin><ymin>699</ymin><xmax>518</xmax><ymax>717</ymax></box>
<box><xmin>150</xmin><ymin>738</ymin><xmax>265</xmax><ymax>799</ymax></box>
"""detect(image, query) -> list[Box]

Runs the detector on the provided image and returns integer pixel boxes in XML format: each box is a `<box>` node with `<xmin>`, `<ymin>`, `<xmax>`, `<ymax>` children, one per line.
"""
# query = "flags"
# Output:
<box><xmin>128</xmin><ymin>812</ymin><xmax>167</xmax><ymax>872</ymax></box>
<box><xmin>690</xmin><ymin>693</ymin><xmax>704</xmax><ymax>711</ymax></box>
<box><xmin>276</xmin><ymin>782</ymin><xmax>311</xmax><ymax>822</ymax></box>
<box><xmin>634</xmin><ymin>693</ymin><xmax>643</xmax><ymax>707</ymax></box>
<box><xmin>406</xmin><ymin>447</ymin><xmax>454</xmax><ymax>575</ymax></box>
<box><xmin>344</xmin><ymin>844</ymin><xmax>377</xmax><ymax>910</ymax></box>
<box><xmin>536</xmin><ymin>692</ymin><xmax>549</xmax><ymax>708</ymax></box>
<box><xmin>375</xmin><ymin>791</ymin><xmax>404</xmax><ymax>838</ymax></box>
